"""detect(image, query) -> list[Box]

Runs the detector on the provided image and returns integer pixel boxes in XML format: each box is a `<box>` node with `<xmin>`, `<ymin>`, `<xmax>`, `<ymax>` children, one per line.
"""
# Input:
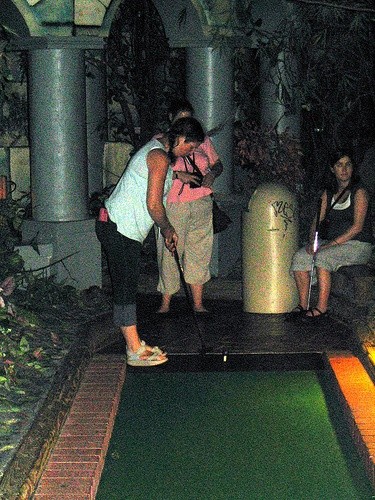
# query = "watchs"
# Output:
<box><xmin>173</xmin><ymin>171</ymin><xmax>179</xmax><ymax>179</ymax></box>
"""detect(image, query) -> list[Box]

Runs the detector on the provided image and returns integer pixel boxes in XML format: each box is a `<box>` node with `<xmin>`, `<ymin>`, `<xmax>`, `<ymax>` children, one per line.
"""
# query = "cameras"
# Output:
<box><xmin>190</xmin><ymin>172</ymin><xmax>202</xmax><ymax>188</ymax></box>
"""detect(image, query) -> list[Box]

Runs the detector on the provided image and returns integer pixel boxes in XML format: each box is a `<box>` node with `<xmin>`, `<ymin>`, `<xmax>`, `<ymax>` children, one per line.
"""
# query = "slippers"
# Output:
<box><xmin>291</xmin><ymin>304</ymin><xmax>308</xmax><ymax>314</ymax></box>
<box><xmin>304</xmin><ymin>307</ymin><xmax>329</xmax><ymax>321</ymax></box>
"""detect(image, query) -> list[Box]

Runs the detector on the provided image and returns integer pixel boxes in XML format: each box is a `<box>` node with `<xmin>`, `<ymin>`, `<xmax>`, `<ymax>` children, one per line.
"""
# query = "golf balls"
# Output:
<box><xmin>222</xmin><ymin>350</ymin><xmax>229</xmax><ymax>355</ymax></box>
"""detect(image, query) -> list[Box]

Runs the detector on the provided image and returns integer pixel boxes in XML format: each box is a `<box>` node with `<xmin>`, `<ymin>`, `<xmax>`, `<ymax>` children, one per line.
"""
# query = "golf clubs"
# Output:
<box><xmin>171</xmin><ymin>239</ymin><xmax>207</xmax><ymax>355</ymax></box>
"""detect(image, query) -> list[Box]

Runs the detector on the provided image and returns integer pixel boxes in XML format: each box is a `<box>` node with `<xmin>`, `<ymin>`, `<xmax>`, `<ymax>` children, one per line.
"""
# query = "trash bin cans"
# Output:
<box><xmin>240</xmin><ymin>182</ymin><xmax>304</xmax><ymax>314</ymax></box>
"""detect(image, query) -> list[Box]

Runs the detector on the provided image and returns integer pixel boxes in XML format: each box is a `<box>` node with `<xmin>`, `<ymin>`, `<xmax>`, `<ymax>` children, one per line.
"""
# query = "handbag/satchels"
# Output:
<box><xmin>212</xmin><ymin>201</ymin><xmax>232</xmax><ymax>234</ymax></box>
<box><xmin>318</xmin><ymin>216</ymin><xmax>330</xmax><ymax>241</ymax></box>
<box><xmin>88</xmin><ymin>184</ymin><xmax>116</xmax><ymax>218</ymax></box>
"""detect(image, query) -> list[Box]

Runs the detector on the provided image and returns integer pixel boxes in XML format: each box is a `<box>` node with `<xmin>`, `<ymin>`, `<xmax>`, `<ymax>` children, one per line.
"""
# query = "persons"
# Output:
<box><xmin>94</xmin><ymin>115</ymin><xmax>206</xmax><ymax>373</ymax></box>
<box><xmin>148</xmin><ymin>98</ymin><xmax>223</xmax><ymax>320</ymax></box>
<box><xmin>288</xmin><ymin>152</ymin><xmax>374</xmax><ymax>318</ymax></box>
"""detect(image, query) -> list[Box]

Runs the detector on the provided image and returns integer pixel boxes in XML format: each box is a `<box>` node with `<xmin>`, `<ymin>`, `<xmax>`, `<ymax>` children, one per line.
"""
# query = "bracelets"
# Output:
<box><xmin>333</xmin><ymin>237</ymin><xmax>340</xmax><ymax>245</ymax></box>
<box><xmin>159</xmin><ymin>222</ymin><xmax>173</xmax><ymax>234</ymax></box>
<box><xmin>155</xmin><ymin>217</ymin><xmax>168</xmax><ymax>226</ymax></box>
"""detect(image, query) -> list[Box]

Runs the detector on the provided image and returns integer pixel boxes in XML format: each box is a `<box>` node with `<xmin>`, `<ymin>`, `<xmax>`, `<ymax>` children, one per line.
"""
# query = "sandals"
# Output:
<box><xmin>126</xmin><ymin>347</ymin><xmax>168</xmax><ymax>367</ymax></box>
<box><xmin>125</xmin><ymin>340</ymin><xmax>167</xmax><ymax>357</ymax></box>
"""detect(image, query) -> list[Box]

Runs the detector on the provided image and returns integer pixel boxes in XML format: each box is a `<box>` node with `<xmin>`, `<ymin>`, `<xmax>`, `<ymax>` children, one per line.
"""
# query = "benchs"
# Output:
<box><xmin>331</xmin><ymin>264</ymin><xmax>375</xmax><ymax>308</ymax></box>
